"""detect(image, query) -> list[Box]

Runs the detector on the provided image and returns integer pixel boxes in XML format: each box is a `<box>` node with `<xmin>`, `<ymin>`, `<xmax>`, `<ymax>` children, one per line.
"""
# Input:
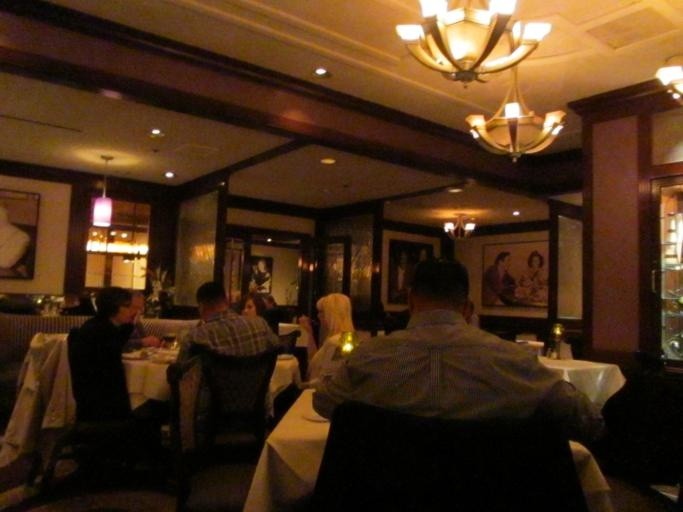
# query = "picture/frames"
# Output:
<box><xmin>0</xmin><ymin>188</ymin><xmax>42</xmax><ymax>280</ymax></box>
<box><xmin>387</xmin><ymin>238</ymin><xmax>436</xmax><ymax>305</ymax></box>
<box><xmin>481</xmin><ymin>240</ymin><xmax>549</xmax><ymax>308</ymax></box>
<box><xmin>249</xmin><ymin>255</ymin><xmax>273</xmax><ymax>295</ymax></box>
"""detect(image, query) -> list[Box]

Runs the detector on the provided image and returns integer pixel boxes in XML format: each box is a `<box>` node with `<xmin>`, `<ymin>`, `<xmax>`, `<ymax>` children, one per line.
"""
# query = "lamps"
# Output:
<box><xmin>652</xmin><ymin>54</ymin><xmax>682</xmax><ymax>103</ymax></box>
<box><xmin>443</xmin><ymin>213</ymin><xmax>477</xmax><ymax>243</ymax></box>
<box><xmin>396</xmin><ymin>0</ymin><xmax>552</xmax><ymax>88</ymax></box>
<box><xmin>91</xmin><ymin>154</ymin><xmax>114</xmax><ymax>228</ymax></box>
<box><xmin>465</xmin><ymin>65</ymin><xmax>568</xmax><ymax>163</ymax></box>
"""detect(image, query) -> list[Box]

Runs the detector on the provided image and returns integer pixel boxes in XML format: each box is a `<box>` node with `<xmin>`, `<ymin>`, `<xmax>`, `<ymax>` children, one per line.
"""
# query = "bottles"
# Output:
<box><xmin>156</xmin><ymin>328</ymin><xmax>183</xmax><ymax>358</ymax></box>
<box><xmin>30</xmin><ymin>293</ymin><xmax>64</xmax><ymax>314</ymax></box>
<box><xmin>544</xmin><ymin>322</ymin><xmax>567</xmax><ymax>360</ymax></box>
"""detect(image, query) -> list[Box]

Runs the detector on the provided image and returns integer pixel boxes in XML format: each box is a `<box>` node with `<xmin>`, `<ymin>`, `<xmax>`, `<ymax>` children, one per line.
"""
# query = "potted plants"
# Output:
<box><xmin>140</xmin><ymin>260</ymin><xmax>175</xmax><ymax>304</ymax></box>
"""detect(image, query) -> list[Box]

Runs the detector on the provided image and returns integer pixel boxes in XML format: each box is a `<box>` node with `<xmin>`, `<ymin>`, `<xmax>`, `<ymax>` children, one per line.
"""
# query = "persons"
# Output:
<box><xmin>244</xmin><ymin>293</ymin><xmax>276</xmax><ymax>333</ymax></box>
<box><xmin>520</xmin><ymin>251</ymin><xmax>546</xmax><ymax>288</ymax></box>
<box><xmin>483</xmin><ymin>252</ymin><xmax>515</xmax><ymax>303</ymax></box>
<box><xmin>166</xmin><ymin>282</ymin><xmax>280</xmax><ymax>434</ymax></box>
<box><xmin>291</xmin><ymin>292</ymin><xmax>359</xmax><ymax>390</ymax></box>
<box><xmin>312</xmin><ymin>257</ymin><xmax>603</xmax><ymax>437</ymax></box>
<box><xmin>120</xmin><ymin>290</ymin><xmax>159</xmax><ymax>353</ymax></box>
<box><xmin>249</xmin><ymin>259</ymin><xmax>270</xmax><ymax>293</ymax></box>
<box><xmin>70</xmin><ymin>287</ymin><xmax>171</xmax><ymax>468</ymax></box>
<box><xmin>264</xmin><ymin>295</ymin><xmax>283</xmax><ymax>325</ymax></box>
